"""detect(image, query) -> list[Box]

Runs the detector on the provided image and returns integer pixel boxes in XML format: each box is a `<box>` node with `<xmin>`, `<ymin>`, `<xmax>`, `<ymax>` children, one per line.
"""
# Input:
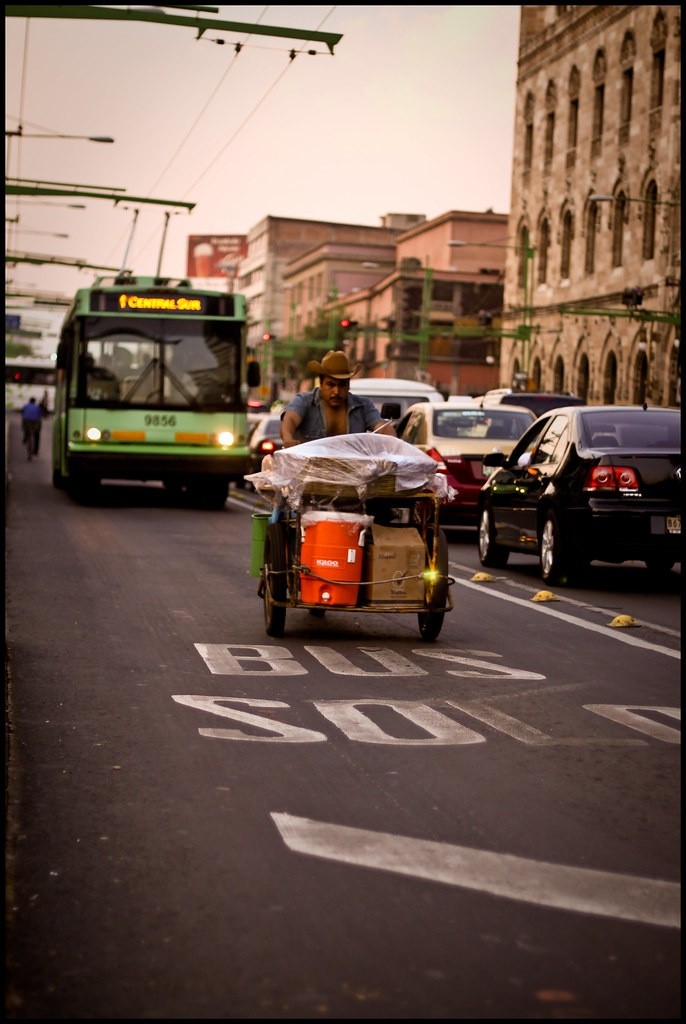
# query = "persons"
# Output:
<box><xmin>20</xmin><ymin>398</ymin><xmax>42</xmax><ymax>455</ymax></box>
<box><xmin>279</xmin><ymin>350</ymin><xmax>397</xmax><ymax>448</ymax></box>
<box><xmin>436</xmin><ymin>380</ymin><xmax>448</xmax><ymax>401</ymax></box>
<box><xmin>484</xmin><ymin>418</ymin><xmax>494</xmax><ymax>433</ymax></box>
<box><xmin>39</xmin><ymin>390</ymin><xmax>53</xmax><ymax>418</ymax></box>
<box><xmin>163</xmin><ymin>350</ymin><xmax>202</xmax><ymax>404</ymax></box>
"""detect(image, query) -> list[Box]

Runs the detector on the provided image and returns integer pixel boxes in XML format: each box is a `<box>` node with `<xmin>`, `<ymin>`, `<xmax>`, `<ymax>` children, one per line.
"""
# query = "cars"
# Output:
<box><xmin>394</xmin><ymin>403</ymin><xmax>539</xmax><ymax>533</ymax></box>
<box><xmin>472</xmin><ymin>388</ymin><xmax>587</xmax><ymax>420</ymax></box>
<box><xmin>476</xmin><ymin>406</ymin><xmax>685</xmax><ymax>586</ymax></box>
<box><xmin>351</xmin><ymin>376</ymin><xmax>446</xmax><ymax>421</ymax></box>
<box><xmin>236</xmin><ymin>416</ymin><xmax>282</xmax><ymax>489</ymax></box>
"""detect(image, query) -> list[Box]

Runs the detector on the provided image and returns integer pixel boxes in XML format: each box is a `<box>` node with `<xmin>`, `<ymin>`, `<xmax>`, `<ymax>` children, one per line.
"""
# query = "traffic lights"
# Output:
<box><xmin>263</xmin><ymin>332</ymin><xmax>277</xmax><ymax>343</ymax></box>
<box><xmin>342</xmin><ymin>318</ymin><xmax>359</xmax><ymax>327</ymax></box>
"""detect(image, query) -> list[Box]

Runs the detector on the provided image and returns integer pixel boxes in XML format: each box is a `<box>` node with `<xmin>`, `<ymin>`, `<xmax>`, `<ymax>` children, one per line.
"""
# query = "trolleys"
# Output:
<box><xmin>251</xmin><ymin>433</ymin><xmax>450</xmax><ymax>639</ymax></box>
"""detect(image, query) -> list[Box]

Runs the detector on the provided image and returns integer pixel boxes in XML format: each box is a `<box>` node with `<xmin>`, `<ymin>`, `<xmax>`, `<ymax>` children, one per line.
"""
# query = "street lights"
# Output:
<box><xmin>362</xmin><ymin>253</ymin><xmax>433</xmax><ymax>381</ymax></box>
<box><xmin>281</xmin><ymin>280</ymin><xmax>340</xmax><ymax>351</ymax></box>
<box><xmin>448</xmin><ymin>239</ymin><xmax>537</xmax><ymax>386</ymax></box>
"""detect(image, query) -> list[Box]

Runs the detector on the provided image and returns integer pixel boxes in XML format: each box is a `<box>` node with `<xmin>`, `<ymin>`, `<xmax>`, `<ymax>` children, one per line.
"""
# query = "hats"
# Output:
<box><xmin>307</xmin><ymin>350</ymin><xmax>361</xmax><ymax>379</ymax></box>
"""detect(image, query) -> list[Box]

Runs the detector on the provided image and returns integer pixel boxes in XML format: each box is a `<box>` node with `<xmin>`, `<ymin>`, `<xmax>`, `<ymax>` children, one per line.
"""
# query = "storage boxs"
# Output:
<box><xmin>360</xmin><ymin>524</ymin><xmax>427</xmax><ymax>606</ymax></box>
<box><xmin>298</xmin><ymin>515</ymin><xmax>373</xmax><ymax>605</ymax></box>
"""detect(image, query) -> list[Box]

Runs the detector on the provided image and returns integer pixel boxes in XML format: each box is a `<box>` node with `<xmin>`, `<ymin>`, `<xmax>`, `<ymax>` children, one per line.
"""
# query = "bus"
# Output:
<box><xmin>53</xmin><ymin>279</ymin><xmax>260</xmax><ymax>512</ymax></box>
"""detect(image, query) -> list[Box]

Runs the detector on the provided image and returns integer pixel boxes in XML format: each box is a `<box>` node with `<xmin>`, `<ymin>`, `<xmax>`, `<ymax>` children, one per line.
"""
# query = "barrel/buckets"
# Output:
<box><xmin>250</xmin><ymin>513</ymin><xmax>272</xmax><ymax>577</ymax></box>
<box><xmin>300</xmin><ymin>511</ymin><xmax>369</xmax><ymax>606</ymax></box>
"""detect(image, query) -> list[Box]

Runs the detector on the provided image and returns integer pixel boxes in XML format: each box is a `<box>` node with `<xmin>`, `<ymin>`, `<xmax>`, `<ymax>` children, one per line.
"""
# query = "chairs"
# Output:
<box><xmin>593</xmin><ymin>436</ymin><xmax>620</xmax><ymax>447</ymax></box>
<box><xmin>486</xmin><ymin>425</ymin><xmax>510</xmax><ymax>437</ymax></box>
<box><xmin>438</xmin><ymin>425</ymin><xmax>456</xmax><ymax>436</ymax></box>
